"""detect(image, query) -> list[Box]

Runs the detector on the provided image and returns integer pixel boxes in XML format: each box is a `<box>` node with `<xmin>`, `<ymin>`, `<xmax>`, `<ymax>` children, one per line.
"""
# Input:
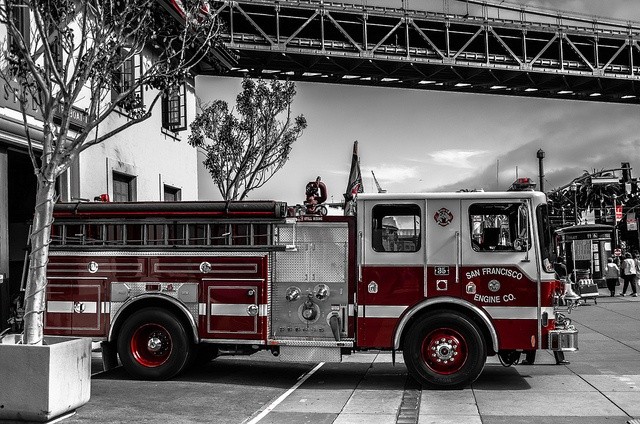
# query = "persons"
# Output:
<box><xmin>520</xmin><ymin>349</ymin><xmax>571</xmax><ymax>365</ymax></box>
<box><xmin>604</xmin><ymin>256</ymin><xmax>621</xmax><ymax>296</ymax></box>
<box><xmin>620</xmin><ymin>252</ymin><xmax>637</xmax><ymax>297</ymax></box>
<box><xmin>553</xmin><ymin>256</ymin><xmax>568</xmax><ymax>278</ymax></box>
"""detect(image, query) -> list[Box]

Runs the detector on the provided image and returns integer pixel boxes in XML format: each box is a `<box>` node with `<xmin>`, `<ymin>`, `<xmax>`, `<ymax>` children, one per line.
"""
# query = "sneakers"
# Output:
<box><xmin>630</xmin><ymin>293</ymin><xmax>638</xmax><ymax>297</ymax></box>
<box><xmin>619</xmin><ymin>292</ymin><xmax>626</xmax><ymax>297</ymax></box>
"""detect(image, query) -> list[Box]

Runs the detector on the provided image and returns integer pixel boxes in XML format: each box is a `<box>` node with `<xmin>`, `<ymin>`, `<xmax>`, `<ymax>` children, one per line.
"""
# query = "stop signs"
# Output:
<box><xmin>614</xmin><ymin>249</ymin><xmax>621</xmax><ymax>256</ymax></box>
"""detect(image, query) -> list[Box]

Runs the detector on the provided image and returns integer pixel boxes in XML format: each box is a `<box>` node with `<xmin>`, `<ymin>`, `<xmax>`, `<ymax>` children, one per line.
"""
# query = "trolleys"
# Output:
<box><xmin>572</xmin><ymin>283</ymin><xmax>599</xmax><ymax>304</ymax></box>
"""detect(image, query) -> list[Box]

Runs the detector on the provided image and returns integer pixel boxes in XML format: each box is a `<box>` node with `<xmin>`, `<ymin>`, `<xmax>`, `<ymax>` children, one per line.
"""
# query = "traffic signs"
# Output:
<box><xmin>616</xmin><ymin>206</ymin><xmax>622</xmax><ymax>219</ymax></box>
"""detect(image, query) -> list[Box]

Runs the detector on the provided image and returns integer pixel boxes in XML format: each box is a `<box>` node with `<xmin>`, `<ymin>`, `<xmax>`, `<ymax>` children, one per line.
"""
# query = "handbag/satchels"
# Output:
<box><xmin>615</xmin><ymin>277</ymin><xmax>620</xmax><ymax>286</ymax></box>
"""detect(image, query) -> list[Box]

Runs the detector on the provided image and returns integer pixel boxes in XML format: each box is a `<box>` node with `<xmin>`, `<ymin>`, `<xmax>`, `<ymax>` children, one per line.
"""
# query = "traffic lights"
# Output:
<box><xmin>623</xmin><ymin>163</ymin><xmax>631</xmax><ymax>182</ymax></box>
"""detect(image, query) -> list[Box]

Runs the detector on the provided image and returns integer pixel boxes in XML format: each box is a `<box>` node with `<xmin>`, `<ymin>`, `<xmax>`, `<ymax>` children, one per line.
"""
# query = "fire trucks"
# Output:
<box><xmin>19</xmin><ymin>176</ymin><xmax>578</xmax><ymax>389</ymax></box>
